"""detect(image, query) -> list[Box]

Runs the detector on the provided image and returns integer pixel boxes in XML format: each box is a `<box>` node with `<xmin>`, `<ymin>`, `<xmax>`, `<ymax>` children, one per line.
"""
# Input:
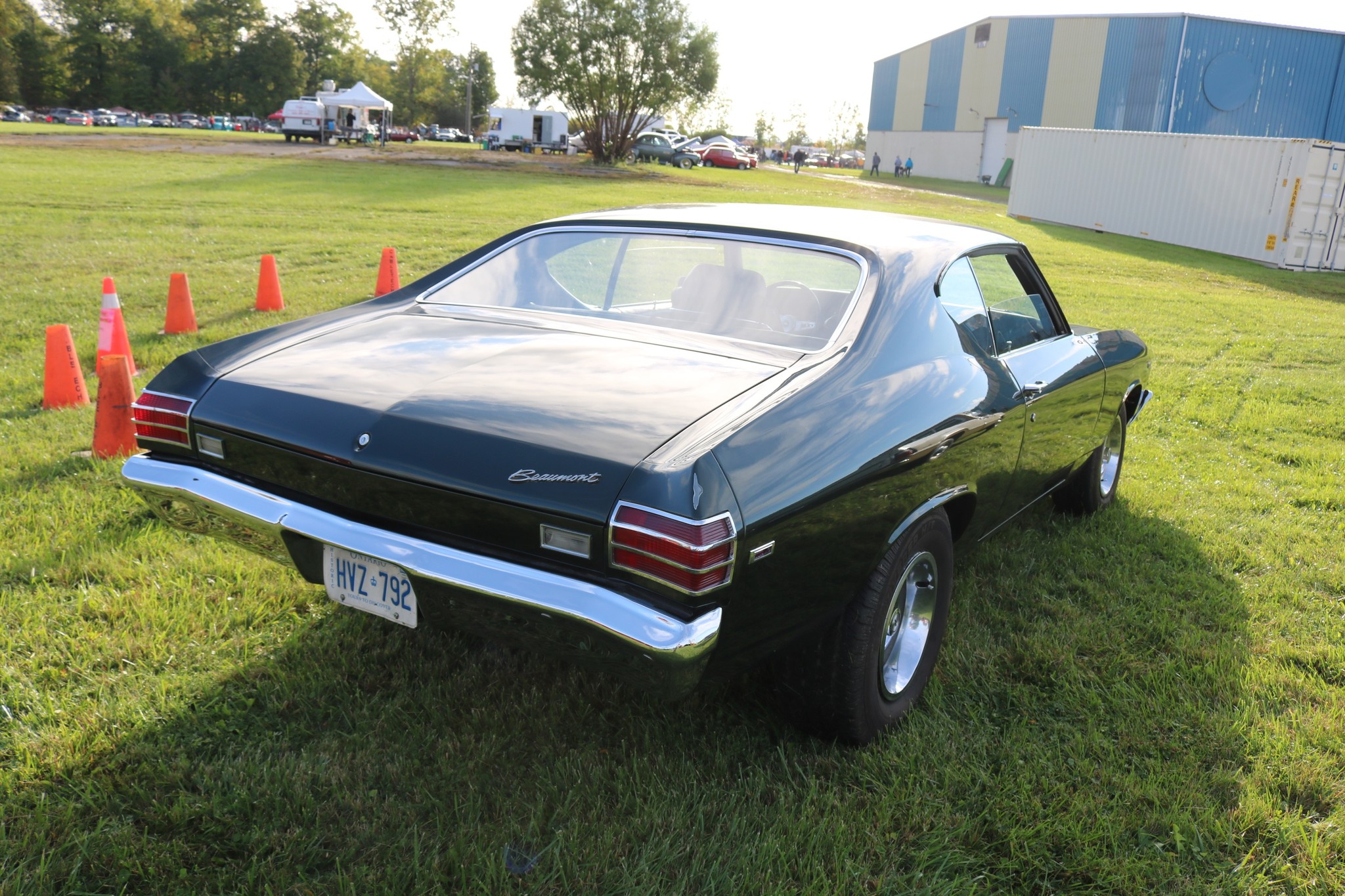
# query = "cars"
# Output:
<box><xmin>805</xmin><ymin>154</ymin><xmax>839</xmax><ymax>168</ymax></box>
<box><xmin>568</xmin><ymin>127</ymin><xmax>757</xmax><ymax>171</ymax></box>
<box><xmin>388</xmin><ymin>122</ymin><xmax>473</xmax><ymax>144</ymax></box>
<box><xmin>0</xmin><ymin>104</ymin><xmax>282</xmax><ymax>134</ymax></box>
<box><xmin>117</xmin><ymin>203</ymin><xmax>1149</xmax><ymax>750</ymax></box>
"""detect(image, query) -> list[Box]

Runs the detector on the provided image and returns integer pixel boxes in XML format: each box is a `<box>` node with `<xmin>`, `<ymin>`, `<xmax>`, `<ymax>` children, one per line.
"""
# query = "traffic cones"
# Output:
<box><xmin>255</xmin><ymin>255</ymin><xmax>285</xmax><ymax>312</ymax></box>
<box><xmin>31</xmin><ymin>324</ymin><xmax>95</xmax><ymax>411</ymax></box>
<box><xmin>90</xmin><ymin>278</ymin><xmax>146</xmax><ymax>378</ymax></box>
<box><xmin>375</xmin><ymin>248</ymin><xmax>400</xmax><ymax>297</ymax></box>
<box><xmin>69</xmin><ymin>355</ymin><xmax>139</xmax><ymax>465</ymax></box>
<box><xmin>158</xmin><ymin>273</ymin><xmax>199</xmax><ymax>335</ymax></box>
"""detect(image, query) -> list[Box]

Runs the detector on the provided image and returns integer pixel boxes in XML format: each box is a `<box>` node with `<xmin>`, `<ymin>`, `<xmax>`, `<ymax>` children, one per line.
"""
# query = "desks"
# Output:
<box><xmin>340</xmin><ymin>127</ymin><xmax>369</xmax><ymax>145</ymax></box>
<box><xmin>533</xmin><ymin>142</ymin><xmax>563</xmax><ymax>155</ymax></box>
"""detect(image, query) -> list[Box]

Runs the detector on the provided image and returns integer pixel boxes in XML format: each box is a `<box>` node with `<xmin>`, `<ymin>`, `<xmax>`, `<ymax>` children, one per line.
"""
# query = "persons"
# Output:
<box><xmin>869</xmin><ymin>151</ymin><xmax>881</xmax><ymax>176</ymax></box>
<box><xmin>826</xmin><ymin>154</ymin><xmax>863</xmax><ymax>168</ymax></box>
<box><xmin>134</xmin><ymin>112</ymin><xmax>138</xmax><ymax>126</ymax></box>
<box><xmin>793</xmin><ymin>149</ymin><xmax>801</xmax><ymax>174</ymax></box>
<box><xmin>894</xmin><ymin>155</ymin><xmax>902</xmax><ymax>178</ymax></box>
<box><xmin>749</xmin><ymin>147</ymin><xmax>792</xmax><ymax>166</ymax></box>
<box><xmin>209</xmin><ymin>115</ymin><xmax>216</xmax><ymax>129</ymax></box>
<box><xmin>904</xmin><ymin>158</ymin><xmax>914</xmax><ymax>178</ymax></box>
<box><xmin>379</xmin><ymin>113</ymin><xmax>384</xmax><ymax>143</ymax></box>
<box><xmin>346</xmin><ymin>109</ymin><xmax>356</xmax><ymax>145</ymax></box>
<box><xmin>899</xmin><ymin>167</ymin><xmax>907</xmax><ymax>178</ymax></box>
<box><xmin>222</xmin><ymin>115</ymin><xmax>267</xmax><ymax>134</ymax></box>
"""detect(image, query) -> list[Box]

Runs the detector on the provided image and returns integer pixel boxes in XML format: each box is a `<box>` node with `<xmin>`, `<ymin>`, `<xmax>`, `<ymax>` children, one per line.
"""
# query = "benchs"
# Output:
<box><xmin>768</xmin><ymin>286</ymin><xmax>1038</xmax><ymax>356</ymax></box>
<box><xmin>336</xmin><ymin>136</ymin><xmax>362</xmax><ymax>141</ymax></box>
<box><xmin>527</xmin><ymin>145</ymin><xmax>568</xmax><ymax>151</ymax></box>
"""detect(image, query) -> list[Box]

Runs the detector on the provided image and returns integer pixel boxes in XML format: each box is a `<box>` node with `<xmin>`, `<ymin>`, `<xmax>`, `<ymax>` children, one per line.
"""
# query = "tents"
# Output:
<box><xmin>322</xmin><ymin>81</ymin><xmax>394</xmax><ymax>146</ymax></box>
<box><xmin>267</xmin><ymin>109</ymin><xmax>284</xmax><ymax>130</ymax></box>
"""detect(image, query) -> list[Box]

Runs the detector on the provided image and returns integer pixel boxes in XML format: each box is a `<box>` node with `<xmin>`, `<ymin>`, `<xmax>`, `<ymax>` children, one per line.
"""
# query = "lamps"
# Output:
<box><xmin>970</xmin><ymin>108</ymin><xmax>979</xmax><ymax>116</ymax></box>
<box><xmin>1006</xmin><ymin>107</ymin><xmax>1017</xmax><ymax>118</ymax></box>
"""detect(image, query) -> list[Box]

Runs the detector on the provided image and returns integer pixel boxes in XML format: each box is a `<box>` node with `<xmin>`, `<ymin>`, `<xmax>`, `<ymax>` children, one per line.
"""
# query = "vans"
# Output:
<box><xmin>280</xmin><ymin>100</ymin><xmax>325</xmax><ymax>143</ymax></box>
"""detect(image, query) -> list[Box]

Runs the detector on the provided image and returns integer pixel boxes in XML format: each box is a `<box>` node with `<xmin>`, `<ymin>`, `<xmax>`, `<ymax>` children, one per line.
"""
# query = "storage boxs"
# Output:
<box><xmin>1007</xmin><ymin>126</ymin><xmax>1345</xmax><ymax>272</ymax></box>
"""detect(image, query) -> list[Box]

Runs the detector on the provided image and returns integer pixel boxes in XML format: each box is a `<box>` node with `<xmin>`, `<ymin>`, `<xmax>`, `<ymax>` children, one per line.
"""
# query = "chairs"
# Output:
<box><xmin>671</xmin><ymin>263</ymin><xmax>766</xmax><ymax>319</ymax></box>
